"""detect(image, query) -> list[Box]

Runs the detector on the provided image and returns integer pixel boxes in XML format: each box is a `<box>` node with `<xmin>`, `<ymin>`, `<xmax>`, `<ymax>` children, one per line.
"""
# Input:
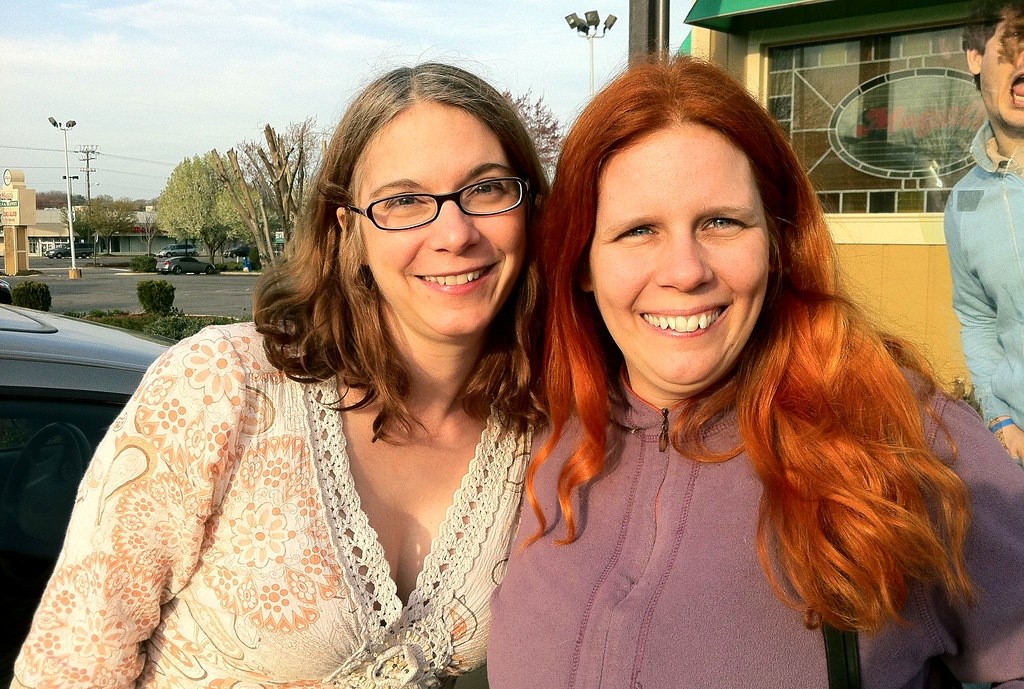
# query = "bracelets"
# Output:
<box><xmin>990</xmin><ymin>417</ymin><xmax>1015</xmax><ymax>434</ymax></box>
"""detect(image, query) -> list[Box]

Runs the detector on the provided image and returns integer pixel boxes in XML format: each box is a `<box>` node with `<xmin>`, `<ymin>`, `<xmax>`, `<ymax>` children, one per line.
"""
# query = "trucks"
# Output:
<box><xmin>46</xmin><ymin>243</ymin><xmax>94</xmax><ymax>259</ymax></box>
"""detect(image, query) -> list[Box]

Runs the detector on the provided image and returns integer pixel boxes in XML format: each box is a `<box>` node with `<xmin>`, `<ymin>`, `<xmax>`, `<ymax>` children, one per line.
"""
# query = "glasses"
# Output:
<box><xmin>348</xmin><ymin>176</ymin><xmax>530</xmax><ymax>232</ymax></box>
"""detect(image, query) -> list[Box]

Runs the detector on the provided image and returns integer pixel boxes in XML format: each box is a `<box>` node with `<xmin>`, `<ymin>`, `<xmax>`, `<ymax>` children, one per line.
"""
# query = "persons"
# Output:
<box><xmin>486</xmin><ymin>55</ymin><xmax>1024</xmax><ymax>689</ymax></box>
<box><xmin>9</xmin><ymin>64</ymin><xmax>550</xmax><ymax>689</ymax></box>
<box><xmin>942</xmin><ymin>0</ymin><xmax>1024</xmax><ymax>465</ymax></box>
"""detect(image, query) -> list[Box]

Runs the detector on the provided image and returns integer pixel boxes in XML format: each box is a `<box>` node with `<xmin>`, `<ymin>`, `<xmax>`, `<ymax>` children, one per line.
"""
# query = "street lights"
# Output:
<box><xmin>565</xmin><ymin>10</ymin><xmax>617</xmax><ymax>99</ymax></box>
<box><xmin>48</xmin><ymin>115</ymin><xmax>81</xmax><ymax>279</ymax></box>
<box><xmin>62</xmin><ymin>176</ymin><xmax>79</xmax><ymax>252</ymax></box>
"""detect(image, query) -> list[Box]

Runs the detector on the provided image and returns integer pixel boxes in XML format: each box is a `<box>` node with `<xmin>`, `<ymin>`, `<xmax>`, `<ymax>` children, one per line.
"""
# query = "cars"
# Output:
<box><xmin>156</xmin><ymin>244</ymin><xmax>198</xmax><ymax>258</ymax></box>
<box><xmin>223</xmin><ymin>245</ymin><xmax>251</xmax><ymax>258</ymax></box>
<box><xmin>155</xmin><ymin>257</ymin><xmax>216</xmax><ymax>275</ymax></box>
<box><xmin>0</xmin><ymin>301</ymin><xmax>183</xmax><ymax>688</ymax></box>
